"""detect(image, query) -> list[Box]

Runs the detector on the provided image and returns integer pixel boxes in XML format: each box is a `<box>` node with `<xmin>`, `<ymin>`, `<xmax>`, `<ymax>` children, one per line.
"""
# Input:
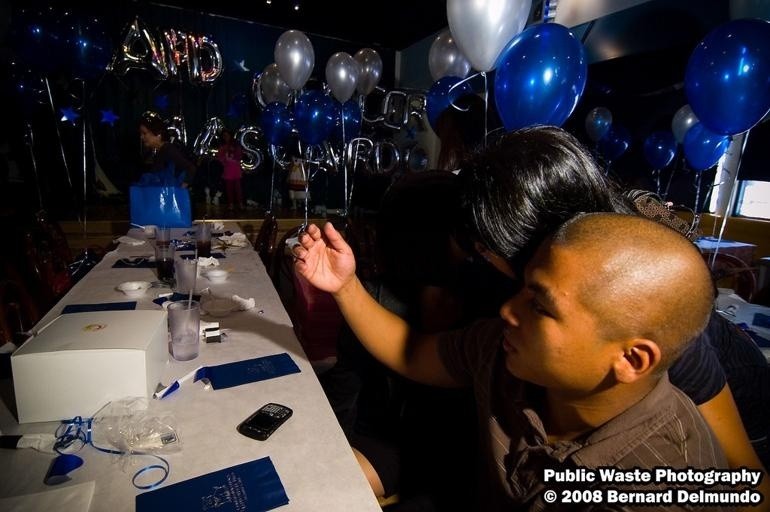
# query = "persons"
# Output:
<box><xmin>429</xmin><ymin>122</ymin><xmax>770</xmax><ymax>512</ymax></box>
<box><xmin>131</xmin><ymin>112</ymin><xmax>208</xmax><ymax>220</ymax></box>
<box><xmin>289</xmin><ymin>209</ymin><xmax>739</xmax><ymax>512</ymax></box>
<box><xmin>319</xmin><ymin>166</ymin><xmax>481</xmax><ymax>448</ymax></box>
<box><xmin>172</xmin><ymin>130</ymin><xmax>395</xmax><ymax>216</ymax></box>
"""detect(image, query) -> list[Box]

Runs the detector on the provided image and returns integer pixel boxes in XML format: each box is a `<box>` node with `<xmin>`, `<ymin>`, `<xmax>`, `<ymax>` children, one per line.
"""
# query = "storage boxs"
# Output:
<box><xmin>10</xmin><ymin>310</ymin><xmax>169</xmax><ymax>424</ymax></box>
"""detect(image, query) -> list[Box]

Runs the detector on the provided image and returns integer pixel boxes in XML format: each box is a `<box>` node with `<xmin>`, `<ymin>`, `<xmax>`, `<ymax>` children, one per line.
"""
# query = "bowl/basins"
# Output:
<box><xmin>117</xmin><ymin>281</ymin><xmax>152</xmax><ymax>297</ymax></box>
<box><xmin>207</xmin><ymin>270</ymin><xmax>229</xmax><ymax>282</ymax></box>
<box><xmin>203</xmin><ymin>300</ymin><xmax>235</xmax><ymax>318</ymax></box>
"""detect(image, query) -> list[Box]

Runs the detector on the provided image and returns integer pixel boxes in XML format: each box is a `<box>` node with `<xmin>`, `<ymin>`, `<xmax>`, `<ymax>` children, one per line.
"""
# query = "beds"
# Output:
<box><xmin>0</xmin><ymin>223</ymin><xmax>383</xmax><ymax>512</ymax></box>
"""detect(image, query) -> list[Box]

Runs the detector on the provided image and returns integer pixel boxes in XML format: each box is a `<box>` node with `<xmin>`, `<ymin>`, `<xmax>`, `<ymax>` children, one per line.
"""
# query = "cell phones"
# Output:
<box><xmin>237</xmin><ymin>403</ymin><xmax>293</xmax><ymax>441</ymax></box>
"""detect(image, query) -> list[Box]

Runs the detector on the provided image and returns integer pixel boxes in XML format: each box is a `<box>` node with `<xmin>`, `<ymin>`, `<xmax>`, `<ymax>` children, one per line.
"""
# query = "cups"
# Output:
<box><xmin>167</xmin><ymin>300</ymin><xmax>201</xmax><ymax>361</ymax></box>
<box><xmin>155</xmin><ymin>223</ymin><xmax>211</xmax><ymax>295</ymax></box>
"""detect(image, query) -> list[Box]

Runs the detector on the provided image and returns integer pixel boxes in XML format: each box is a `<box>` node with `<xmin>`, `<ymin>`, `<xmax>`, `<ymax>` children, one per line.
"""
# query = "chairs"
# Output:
<box><xmin>703</xmin><ymin>253</ymin><xmax>757</xmax><ymax>304</ymax></box>
<box><xmin>0</xmin><ymin>214</ymin><xmax>308</xmax><ymax>345</ymax></box>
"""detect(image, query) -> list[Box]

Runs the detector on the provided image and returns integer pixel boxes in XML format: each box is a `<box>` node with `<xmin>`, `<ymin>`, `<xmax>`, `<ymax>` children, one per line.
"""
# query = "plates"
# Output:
<box><xmin>223</xmin><ymin>245</ymin><xmax>242</xmax><ymax>250</ymax></box>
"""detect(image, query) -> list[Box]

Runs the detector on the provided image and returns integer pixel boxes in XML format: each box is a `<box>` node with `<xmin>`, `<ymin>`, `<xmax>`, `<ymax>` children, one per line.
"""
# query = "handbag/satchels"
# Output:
<box><xmin>129</xmin><ymin>186</ymin><xmax>193</xmax><ymax>228</ymax></box>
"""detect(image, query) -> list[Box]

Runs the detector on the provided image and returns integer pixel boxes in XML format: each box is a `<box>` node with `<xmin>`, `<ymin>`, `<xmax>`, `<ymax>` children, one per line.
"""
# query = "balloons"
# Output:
<box><xmin>247</xmin><ymin>0</ymin><xmax>769</xmax><ymax>171</ymax></box>
